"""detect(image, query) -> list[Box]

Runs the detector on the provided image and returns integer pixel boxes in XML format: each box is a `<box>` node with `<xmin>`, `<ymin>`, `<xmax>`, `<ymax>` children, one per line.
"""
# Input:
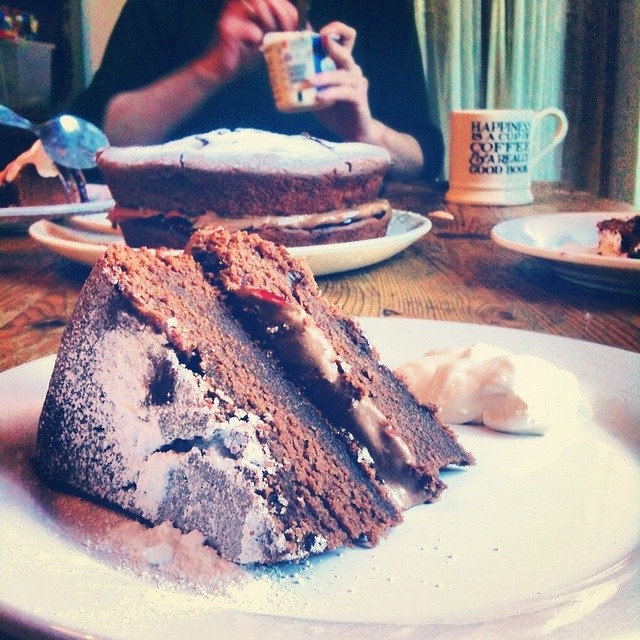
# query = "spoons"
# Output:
<box><xmin>0</xmin><ymin>104</ymin><xmax>110</xmax><ymax>169</ymax></box>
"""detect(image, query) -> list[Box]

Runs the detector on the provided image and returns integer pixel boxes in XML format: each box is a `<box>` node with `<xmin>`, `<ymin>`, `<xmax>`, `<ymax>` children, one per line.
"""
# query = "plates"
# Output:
<box><xmin>1</xmin><ymin>184</ymin><xmax>112</xmax><ymax>225</ymax></box>
<box><xmin>28</xmin><ymin>203</ymin><xmax>433</xmax><ymax>278</ymax></box>
<box><xmin>489</xmin><ymin>210</ymin><xmax>640</xmax><ymax>304</ymax></box>
<box><xmin>0</xmin><ymin>317</ymin><xmax>638</xmax><ymax>636</ymax></box>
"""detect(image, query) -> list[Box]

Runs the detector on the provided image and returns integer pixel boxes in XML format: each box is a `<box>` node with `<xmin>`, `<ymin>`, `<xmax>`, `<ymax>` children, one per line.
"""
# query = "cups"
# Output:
<box><xmin>260</xmin><ymin>33</ymin><xmax>337</xmax><ymax>115</ymax></box>
<box><xmin>445</xmin><ymin>107</ymin><xmax>569</xmax><ymax>208</ymax></box>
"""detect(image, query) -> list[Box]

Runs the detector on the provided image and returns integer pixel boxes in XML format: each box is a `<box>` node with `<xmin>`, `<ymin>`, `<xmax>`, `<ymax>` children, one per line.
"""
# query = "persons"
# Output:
<box><xmin>72</xmin><ymin>0</ymin><xmax>446</xmax><ymax>182</ymax></box>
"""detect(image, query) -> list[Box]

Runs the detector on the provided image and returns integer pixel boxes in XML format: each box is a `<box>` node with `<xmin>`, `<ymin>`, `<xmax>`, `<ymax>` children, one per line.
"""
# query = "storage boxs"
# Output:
<box><xmin>0</xmin><ymin>38</ymin><xmax>56</xmax><ymax>122</ymax></box>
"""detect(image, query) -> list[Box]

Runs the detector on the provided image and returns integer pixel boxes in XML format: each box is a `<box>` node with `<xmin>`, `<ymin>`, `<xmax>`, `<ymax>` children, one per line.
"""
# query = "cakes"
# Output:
<box><xmin>13</xmin><ymin>162</ymin><xmax>80</xmax><ymax>206</ymax></box>
<box><xmin>24</xmin><ymin>243</ymin><xmax>407</xmax><ymax>566</ymax></box>
<box><xmin>597</xmin><ymin>212</ymin><xmax>639</xmax><ymax>257</ymax></box>
<box><xmin>181</xmin><ymin>228</ymin><xmax>475</xmax><ymax>511</ymax></box>
<box><xmin>63</xmin><ymin>164</ymin><xmax>90</xmax><ymax>204</ymax></box>
<box><xmin>95</xmin><ymin>127</ymin><xmax>394</xmax><ymax>246</ymax></box>
